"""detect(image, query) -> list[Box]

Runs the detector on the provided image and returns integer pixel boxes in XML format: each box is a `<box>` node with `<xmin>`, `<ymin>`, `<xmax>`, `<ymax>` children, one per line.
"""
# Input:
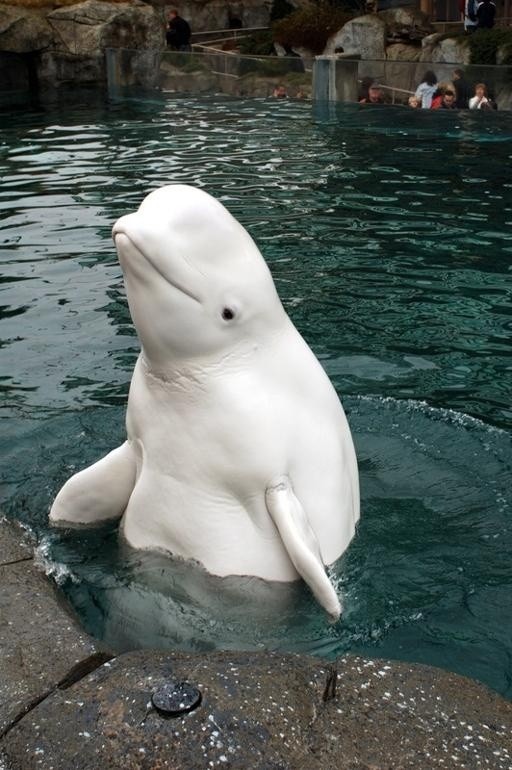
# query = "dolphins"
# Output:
<box><xmin>46</xmin><ymin>184</ymin><xmax>361</xmax><ymax>654</ymax></box>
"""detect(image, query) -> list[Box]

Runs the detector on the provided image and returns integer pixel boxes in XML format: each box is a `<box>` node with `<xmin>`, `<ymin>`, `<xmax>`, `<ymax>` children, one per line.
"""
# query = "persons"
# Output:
<box><xmin>464</xmin><ymin>0</ymin><xmax>497</xmax><ymax>35</ymax></box>
<box><xmin>164</xmin><ymin>9</ymin><xmax>192</xmax><ymax>53</ymax></box>
<box><xmin>268</xmin><ymin>84</ymin><xmax>291</xmax><ymax>99</ymax></box>
<box><xmin>356</xmin><ymin>68</ymin><xmax>498</xmax><ymax>111</ymax></box>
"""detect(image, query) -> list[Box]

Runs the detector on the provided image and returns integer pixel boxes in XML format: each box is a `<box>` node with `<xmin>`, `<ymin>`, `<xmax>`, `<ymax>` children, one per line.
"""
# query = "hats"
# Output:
<box><xmin>369</xmin><ymin>81</ymin><xmax>381</xmax><ymax>88</ymax></box>
